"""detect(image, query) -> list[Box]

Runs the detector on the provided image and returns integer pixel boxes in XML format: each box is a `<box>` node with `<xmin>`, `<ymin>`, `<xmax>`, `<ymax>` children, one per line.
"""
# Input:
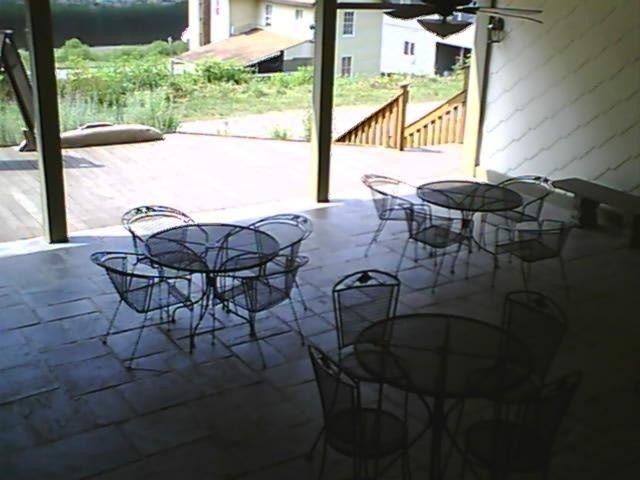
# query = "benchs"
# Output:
<box><xmin>551</xmin><ymin>177</ymin><xmax>640</xmax><ymax>247</ymax></box>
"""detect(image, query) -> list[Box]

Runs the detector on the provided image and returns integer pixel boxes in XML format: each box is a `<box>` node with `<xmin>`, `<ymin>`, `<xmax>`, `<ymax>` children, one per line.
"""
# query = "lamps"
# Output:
<box><xmin>418</xmin><ymin>14</ymin><xmax>471</xmax><ymax>36</ymax></box>
<box><xmin>487</xmin><ymin>17</ymin><xmax>505</xmax><ymax>43</ymax></box>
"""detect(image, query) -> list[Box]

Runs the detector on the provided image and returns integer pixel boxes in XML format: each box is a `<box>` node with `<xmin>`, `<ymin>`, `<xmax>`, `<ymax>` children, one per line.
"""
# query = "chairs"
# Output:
<box><xmin>478</xmin><ymin>174</ymin><xmax>556</xmax><ymax>247</ymax></box>
<box><xmin>466</xmin><ymin>378</ymin><xmax>583</xmax><ymax>480</ymax></box>
<box><xmin>215</xmin><ymin>253</ymin><xmax>310</xmax><ymax>369</ymax></box>
<box><xmin>90</xmin><ymin>250</ymin><xmax>194</xmax><ymax>369</ymax></box>
<box><xmin>121</xmin><ymin>204</ymin><xmax>209</xmax><ymax>314</ymax></box>
<box><xmin>307</xmin><ymin>344</ymin><xmax>410</xmax><ymax>480</ymax></box>
<box><xmin>359</xmin><ymin>172</ymin><xmax>426</xmax><ymax>258</ymax></box>
<box><xmin>214</xmin><ymin>214</ymin><xmax>315</xmax><ymax>312</ymax></box>
<box><xmin>395</xmin><ymin>201</ymin><xmax>475</xmax><ymax>296</ymax></box>
<box><xmin>454</xmin><ymin>289</ymin><xmax>569</xmax><ymax>426</ymax></box>
<box><xmin>330</xmin><ymin>268</ymin><xmax>409</xmax><ymax>420</ymax></box>
<box><xmin>493</xmin><ymin>217</ymin><xmax>570</xmax><ymax>302</ymax></box>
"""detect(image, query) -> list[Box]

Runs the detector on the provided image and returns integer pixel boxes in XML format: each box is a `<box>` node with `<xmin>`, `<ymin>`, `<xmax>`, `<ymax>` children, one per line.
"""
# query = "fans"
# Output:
<box><xmin>330</xmin><ymin>0</ymin><xmax>543</xmax><ymax>21</ymax></box>
